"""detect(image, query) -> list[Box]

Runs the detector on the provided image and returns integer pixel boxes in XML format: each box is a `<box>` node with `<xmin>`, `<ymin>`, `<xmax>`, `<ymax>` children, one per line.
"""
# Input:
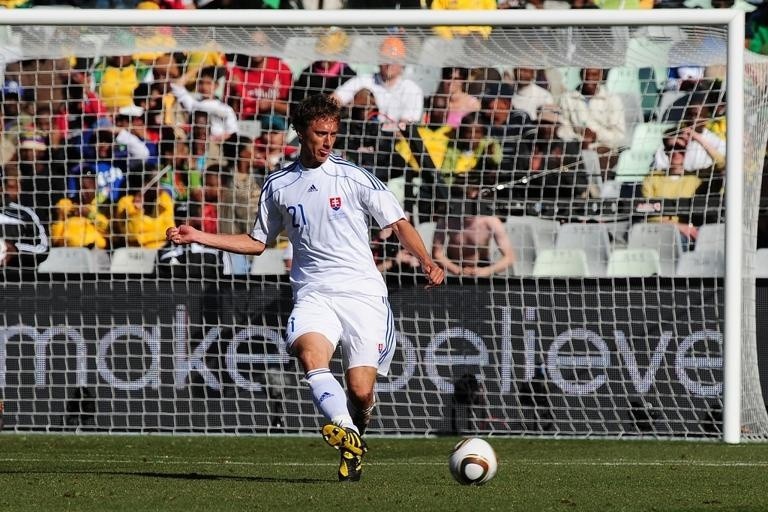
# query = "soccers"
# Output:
<box><xmin>449</xmin><ymin>437</ymin><xmax>498</xmax><ymax>485</ymax></box>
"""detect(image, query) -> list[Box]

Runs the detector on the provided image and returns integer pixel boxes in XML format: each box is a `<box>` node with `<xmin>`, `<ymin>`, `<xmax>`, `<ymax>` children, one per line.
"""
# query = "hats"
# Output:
<box><xmin>379</xmin><ymin>37</ymin><xmax>405</xmax><ymax>57</ymax></box>
<box><xmin>315</xmin><ymin>26</ymin><xmax>348</xmax><ymax>55</ymax></box>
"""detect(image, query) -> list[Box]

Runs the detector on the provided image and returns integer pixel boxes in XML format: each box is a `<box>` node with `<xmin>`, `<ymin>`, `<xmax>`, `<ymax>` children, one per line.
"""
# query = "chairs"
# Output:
<box><xmin>38</xmin><ymin>33</ymin><xmax>726</xmax><ymax>280</ymax></box>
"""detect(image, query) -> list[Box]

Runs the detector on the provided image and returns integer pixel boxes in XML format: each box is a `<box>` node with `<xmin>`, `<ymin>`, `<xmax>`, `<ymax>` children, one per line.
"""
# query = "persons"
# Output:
<box><xmin>164</xmin><ymin>92</ymin><xmax>447</xmax><ymax>484</ymax></box>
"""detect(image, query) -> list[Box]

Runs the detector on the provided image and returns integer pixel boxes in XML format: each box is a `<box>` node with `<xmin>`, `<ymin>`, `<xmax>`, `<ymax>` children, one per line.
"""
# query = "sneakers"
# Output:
<box><xmin>323</xmin><ymin>424</ymin><xmax>368</xmax><ymax>481</ymax></box>
